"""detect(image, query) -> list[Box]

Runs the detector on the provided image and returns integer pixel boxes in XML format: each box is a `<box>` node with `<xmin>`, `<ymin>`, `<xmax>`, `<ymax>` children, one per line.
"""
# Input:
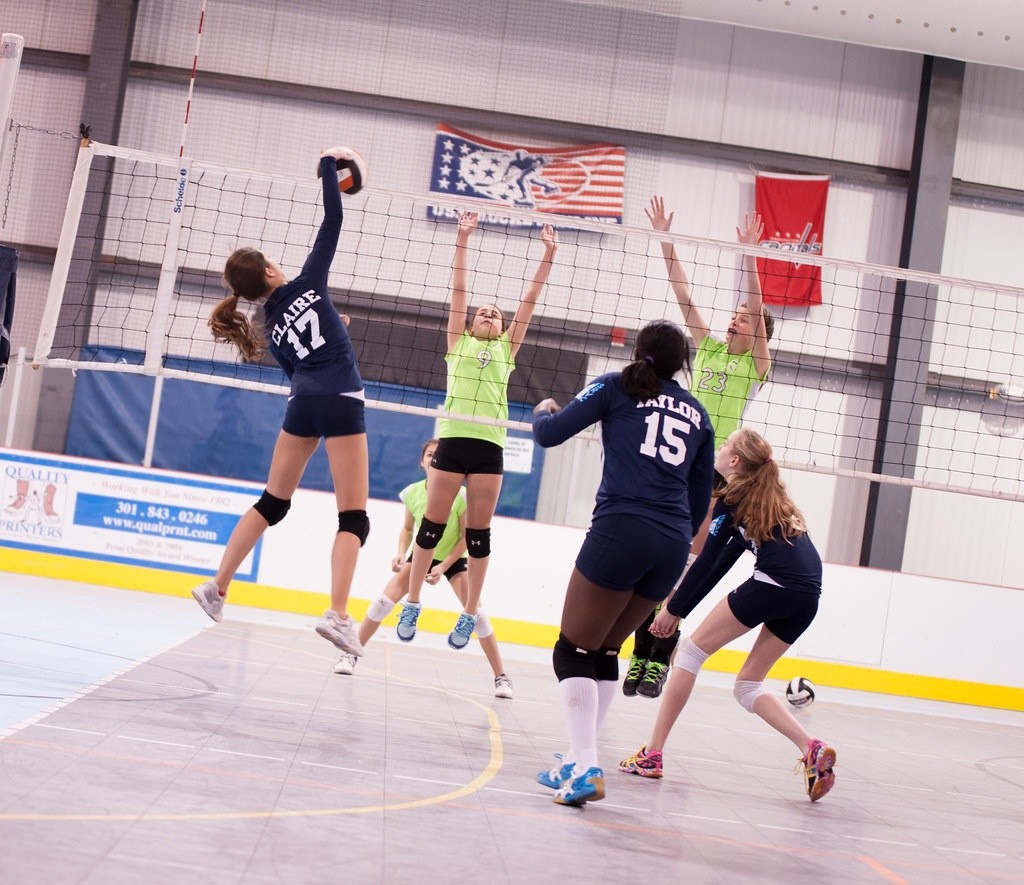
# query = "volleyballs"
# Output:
<box><xmin>316</xmin><ymin>147</ymin><xmax>367</xmax><ymax>199</ymax></box>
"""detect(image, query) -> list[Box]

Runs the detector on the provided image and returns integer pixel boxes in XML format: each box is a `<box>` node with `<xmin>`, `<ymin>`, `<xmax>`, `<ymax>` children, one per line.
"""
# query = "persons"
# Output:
<box><xmin>192</xmin><ymin>151</ymin><xmax>370</xmax><ymax>658</ymax></box>
<box><xmin>622</xmin><ymin>193</ymin><xmax>776</xmax><ymax>701</ymax></box>
<box><xmin>618</xmin><ymin>430</ymin><xmax>838</xmax><ymax>804</ymax></box>
<box><xmin>396</xmin><ymin>209</ymin><xmax>559</xmax><ymax>651</ymax></box>
<box><xmin>532</xmin><ymin>319</ymin><xmax>715</xmax><ymax>806</ymax></box>
<box><xmin>333</xmin><ymin>439</ymin><xmax>518</xmax><ymax>701</ymax></box>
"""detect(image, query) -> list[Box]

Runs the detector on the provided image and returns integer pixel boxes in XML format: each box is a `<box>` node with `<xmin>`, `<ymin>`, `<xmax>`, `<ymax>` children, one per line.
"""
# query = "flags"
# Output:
<box><xmin>422</xmin><ymin>125</ymin><xmax>626</xmax><ymax>231</ymax></box>
<box><xmin>754</xmin><ymin>171</ymin><xmax>837</xmax><ymax>308</ymax></box>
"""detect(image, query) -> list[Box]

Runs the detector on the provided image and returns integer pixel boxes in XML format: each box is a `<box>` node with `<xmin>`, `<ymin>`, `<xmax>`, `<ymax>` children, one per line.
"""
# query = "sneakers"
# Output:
<box><xmin>622</xmin><ymin>656</ymin><xmax>646</xmax><ymax>698</ymax></box>
<box><xmin>495</xmin><ymin>673</ymin><xmax>514</xmax><ymax>698</ymax></box>
<box><xmin>316</xmin><ymin>610</ymin><xmax>365</xmax><ymax>657</ymax></box>
<box><xmin>334</xmin><ymin>652</ymin><xmax>357</xmax><ymax>675</ymax></box>
<box><xmin>801</xmin><ymin>740</ymin><xmax>835</xmax><ymax>803</ymax></box>
<box><xmin>537</xmin><ymin>762</ymin><xmax>575</xmax><ymax>789</ymax></box>
<box><xmin>397</xmin><ymin>600</ymin><xmax>420</xmax><ymax>641</ymax></box>
<box><xmin>448</xmin><ymin>612</ymin><xmax>479</xmax><ymax>650</ymax></box>
<box><xmin>554</xmin><ymin>767</ymin><xmax>605</xmax><ymax>803</ymax></box>
<box><xmin>618</xmin><ymin>744</ymin><xmax>663</xmax><ymax>776</ymax></box>
<box><xmin>192</xmin><ymin>580</ymin><xmax>223</xmax><ymax>624</ymax></box>
<box><xmin>636</xmin><ymin>659</ymin><xmax>668</xmax><ymax>697</ymax></box>
